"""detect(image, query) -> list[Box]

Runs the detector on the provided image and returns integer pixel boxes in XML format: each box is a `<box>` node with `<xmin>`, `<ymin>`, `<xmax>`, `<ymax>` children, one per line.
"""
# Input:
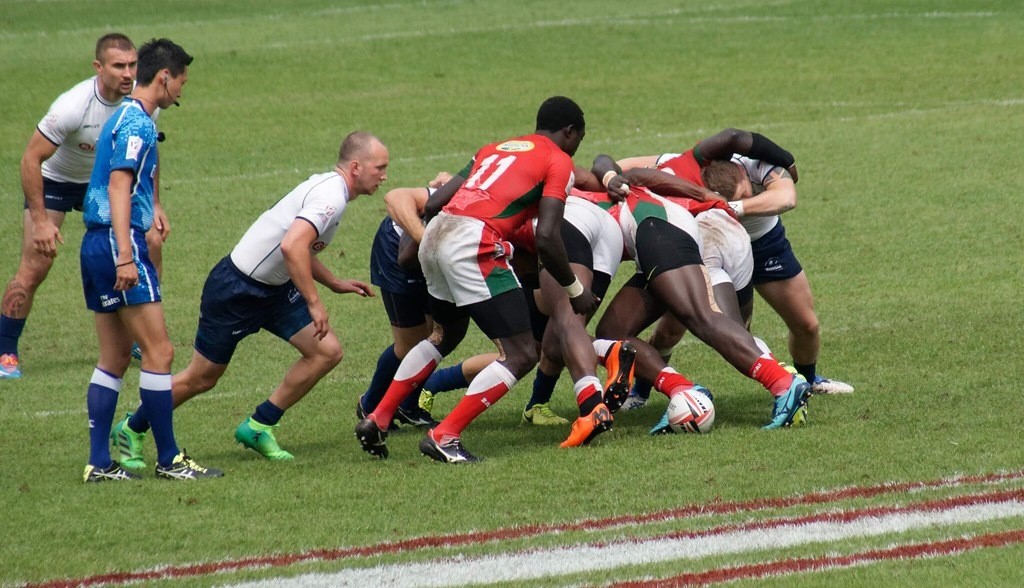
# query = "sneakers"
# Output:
<box><xmin>132</xmin><ymin>343</ymin><xmax>142</xmax><ymax>360</ymax></box>
<box><xmin>0</xmin><ymin>353</ymin><xmax>22</xmax><ymax>378</ymax></box>
<box><xmin>778</xmin><ymin>362</ymin><xmax>809</xmax><ymax>428</ymax></box>
<box><xmin>234</xmin><ymin>416</ymin><xmax>295</xmax><ymax>460</ymax></box>
<box><xmin>418</xmin><ymin>429</ymin><xmax>479</xmax><ymax>464</ymax></box>
<box><xmin>761</xmin><ymin>375</ymin><xmax>813</xmax><ymax>430</ymax></box>
<box><xmin>396</xmin><ymin>406</ymin><xmax>441</xmax><ymax>429</ymax></box>
<box><xmin>154</xmin><ymin>448</ymin><xmax>224</xmax><ymax>479</ymax></box>
<box><xmin>83</xmin><ymin>460</ymin><xmax>143</xmax><ymax>482</ymax></box>
<box><xmin>620</xmin><ymin>384</ymin><xmax>648</xmax><ymax>411</ymax></box>
<box><xmin>520</xmin><ymin>402</ymin><xmax>569</xmax><ymax>425</ymax></box>
<box><xmin>109</xmin><ymin>412</ymin><xmax>147</xmax><ymax>470</ymax></box>
<box><xmin>356</xmin><ymin>394</ymin><xmax>399</xmax><ymax>431</ymax></box>
<box><xmin>418</xmin><ymin>387</ymin><xmax>437</xmax><ymax>413</ymax></box>
<box><xmin>649</xmin><ymin>383</ymin><xmax>713</xmax><ymax>435</ymax></box>
<box><xmin>559</xmin><ymin>403</ymin><xmax>614</xmax><ymax>448</ymax></box>
<box><xmin>810</xmin><ymin>376</ymin><xmax>854</xmax><ymax>395</ymax></box>
<box><xmin>602</xmin><ymin>340</ymin><xmax>637</xmax><ymax>414</ymax></box>
<box><xmin>353</xmin><ymin>414</ymin><xmax>388</xmax><ymax>459</ymax></box>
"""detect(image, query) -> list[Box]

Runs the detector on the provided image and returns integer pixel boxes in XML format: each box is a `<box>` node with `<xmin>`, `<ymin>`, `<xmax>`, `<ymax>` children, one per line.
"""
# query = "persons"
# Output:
<box><xmin>354</xmin><ymin>95</ymin><xmax>587</xmax><ymax>463</ymax></box>
<box><xmin>114</xmin><ymin>132</ymin><xmax>388</xmax><ymax>467</ymax></box>
<box><xmin>0</xmin><ymin>34</ymin><xmax>169</xmax><ymax>378</ymax></box>
<box><xmin>358</xmin><ymin>127</ymin><xmax>854</xmax><ymax>448</ymax></box>
<box><xmin>80</xmin><ymin>40</ymin><xmax>224</xmax><ymax>483</ymax></box>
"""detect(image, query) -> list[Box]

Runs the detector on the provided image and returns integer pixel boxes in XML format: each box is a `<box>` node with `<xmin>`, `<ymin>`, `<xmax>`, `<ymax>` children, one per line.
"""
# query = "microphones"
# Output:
<box><xmin>165</xmin><ymin>82</ymin><xmax>180</xmax><ymax>107</ymax></box>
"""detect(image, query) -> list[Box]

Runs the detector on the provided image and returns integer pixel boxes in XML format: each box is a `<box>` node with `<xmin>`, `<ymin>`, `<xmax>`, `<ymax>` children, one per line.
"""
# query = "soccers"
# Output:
<box><xmin>667</xmin><ymin>389</ymin><xmax>716</xmax><ymax>434</ymax></box>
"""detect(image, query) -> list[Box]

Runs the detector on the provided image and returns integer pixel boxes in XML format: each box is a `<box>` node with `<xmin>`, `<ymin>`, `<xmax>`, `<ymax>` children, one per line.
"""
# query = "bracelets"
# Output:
<box><xmin>602</xmin><ymin>171</ymin><xmax>617</xmax><ymax>187</ymax></box>
<box><xmin>728</xmin><ymin>200</ymin><xmax>744</xmax><ymax>218</ymax></box>
<box><xmin>561</xmin><ymin>275</ymin><xmax>584</xmax><ymax>298</ymax></box>
<box><xmin>116</xmin><ymin>260</ymin><xmax>133</xmax><ymax>269</ymax></box>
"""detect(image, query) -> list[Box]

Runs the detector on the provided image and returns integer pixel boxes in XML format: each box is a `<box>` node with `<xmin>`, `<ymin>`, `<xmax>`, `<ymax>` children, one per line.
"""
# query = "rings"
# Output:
<box><xmin>127</xmin><ymin>284</ymin><xmax>131</xmax><ymax>286</ymax></box>
